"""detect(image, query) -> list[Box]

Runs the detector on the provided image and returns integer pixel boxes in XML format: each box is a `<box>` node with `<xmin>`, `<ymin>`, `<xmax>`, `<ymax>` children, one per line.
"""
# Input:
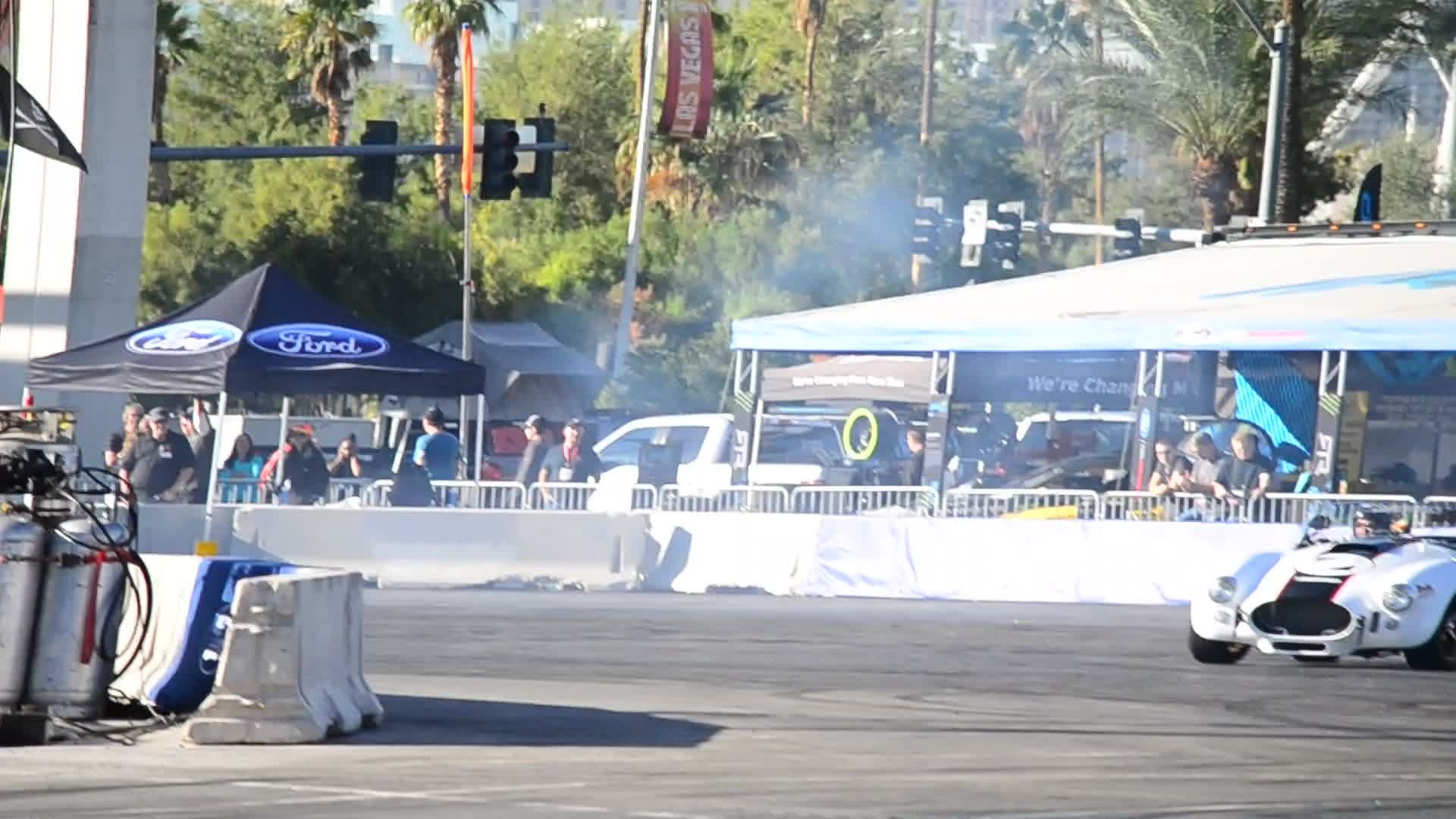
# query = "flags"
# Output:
<box><xmin>0</xmin><ymin>65</ymin><xmax>89</xmax><ymax>175</ymax></box>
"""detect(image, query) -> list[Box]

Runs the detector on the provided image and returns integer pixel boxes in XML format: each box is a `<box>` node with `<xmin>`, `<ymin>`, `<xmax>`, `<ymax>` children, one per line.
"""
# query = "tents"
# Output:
<box><xmin>374</xmin><ymin>318</ymin><xmax>610</xmax><ymax>481</ymax></box>
<box><xmin>747</xmin><ymin>354</ymin><xmax>952</xmax><ymax>513</ymax></box>
<box><xmin>726</xmin><ymin>236</ymin><xmax>1456</xmax><ymax>534</ymax></box>
<box><xmin>12</xmin><ymin>264</ymin><xmax>487</xmax><ymax>555</ymax></box>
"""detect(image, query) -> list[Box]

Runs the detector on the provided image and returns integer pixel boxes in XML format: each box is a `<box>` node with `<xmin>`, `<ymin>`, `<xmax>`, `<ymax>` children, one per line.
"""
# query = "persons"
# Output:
<box><xmin>538</xmin><ymin>416</ymin><xmax>601</xmax><ymax>511</ymax></box>
<box><xmin>1148</xmin><ymin>421</ymin><xmax>1275</xmax><ymax>520</ymax></box>
<box><xmin>900</xmin><ymin>429</ymin><xmax>927</xmax><ymax>504</ymax></box>
<box><xmin>104</xmin><ymin>391</ymin><xmax>363</xmax><ymax>505</ymax></box>
<box><xmin>510</xmin><ymin>413</ymin><xmax>551</xmax><ymax>510</ymax></box>
<box><xmin>1351</xmin><ymin>509</ymin><xmax>1385</xmax><ymax>541</ymax></box>
<box><xmin>408</xmin><ymin>402</ymin><xmax>463</xmax><ymax>506</ymax></box>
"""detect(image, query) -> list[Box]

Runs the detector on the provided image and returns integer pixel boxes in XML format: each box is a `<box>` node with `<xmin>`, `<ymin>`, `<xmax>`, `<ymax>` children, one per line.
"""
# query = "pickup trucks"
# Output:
<box><xmin>588</xmin><ymin>411</ymin><xmax>859</xmax><ymax>515</ymax></box>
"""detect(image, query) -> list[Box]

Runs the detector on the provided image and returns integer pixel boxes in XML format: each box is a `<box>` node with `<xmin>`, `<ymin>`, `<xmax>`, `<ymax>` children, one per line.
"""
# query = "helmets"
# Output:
<box><xmin>1352</xmin><ymin>504</ymin><xmax>1391</xmax><ymax>539</ymax></box>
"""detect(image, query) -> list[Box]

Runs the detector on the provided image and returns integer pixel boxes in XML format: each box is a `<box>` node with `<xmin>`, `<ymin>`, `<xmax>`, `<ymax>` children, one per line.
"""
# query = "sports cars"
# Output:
<box><xmin>1187</xmin><ymin>514</ymin><xmax>1456</xmax><ymax>672</ymax></box>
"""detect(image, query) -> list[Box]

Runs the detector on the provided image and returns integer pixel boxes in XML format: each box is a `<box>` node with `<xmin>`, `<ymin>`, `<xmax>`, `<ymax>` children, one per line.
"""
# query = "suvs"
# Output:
<box><xmin>1014</xmin><ymin>407</ymin><xmax>1219</xmax><ymax>490</ymax></box>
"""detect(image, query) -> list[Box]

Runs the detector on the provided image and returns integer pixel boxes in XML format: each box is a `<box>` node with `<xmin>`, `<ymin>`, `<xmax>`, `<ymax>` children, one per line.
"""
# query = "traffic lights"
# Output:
<box><xmin>986</xmin><ymin>200</ymin><xmax>1025</xmax><ymax>263</ymax></box>
<box><xmin>480</xmin><ymin>117</ymin><xmax>521</xmax><ymax>199</ymax></box>
<box><xmin>909</xmin><ymin>196</ymin><xmax>943</xmax><ymax>262</ymax></box>
<box><xmin>1111</xmin><ymin>207</ymin><xmax>1145</xmax><ymax>261</ymax></box>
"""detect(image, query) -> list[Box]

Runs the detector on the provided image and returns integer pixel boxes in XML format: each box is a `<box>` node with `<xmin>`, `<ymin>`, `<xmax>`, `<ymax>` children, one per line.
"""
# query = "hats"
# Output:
<box><xmin>149</xmin><ymin>406</ymin><xmax>167</xmax><ymax>422</ymax></box>
<box><xmin>519</xmin><ymin>414</ymin><xmax>545</xmax><ymax>430</ymax></box>
<box><xmin>566</xmin><ymin>418</ymin><xmax>585</xmax><ymax>428</ymax></box>
<box><xmin>420</xmin><ymin>406</ymin><xmax>443</xmax><ymax>422</ymax></box>
